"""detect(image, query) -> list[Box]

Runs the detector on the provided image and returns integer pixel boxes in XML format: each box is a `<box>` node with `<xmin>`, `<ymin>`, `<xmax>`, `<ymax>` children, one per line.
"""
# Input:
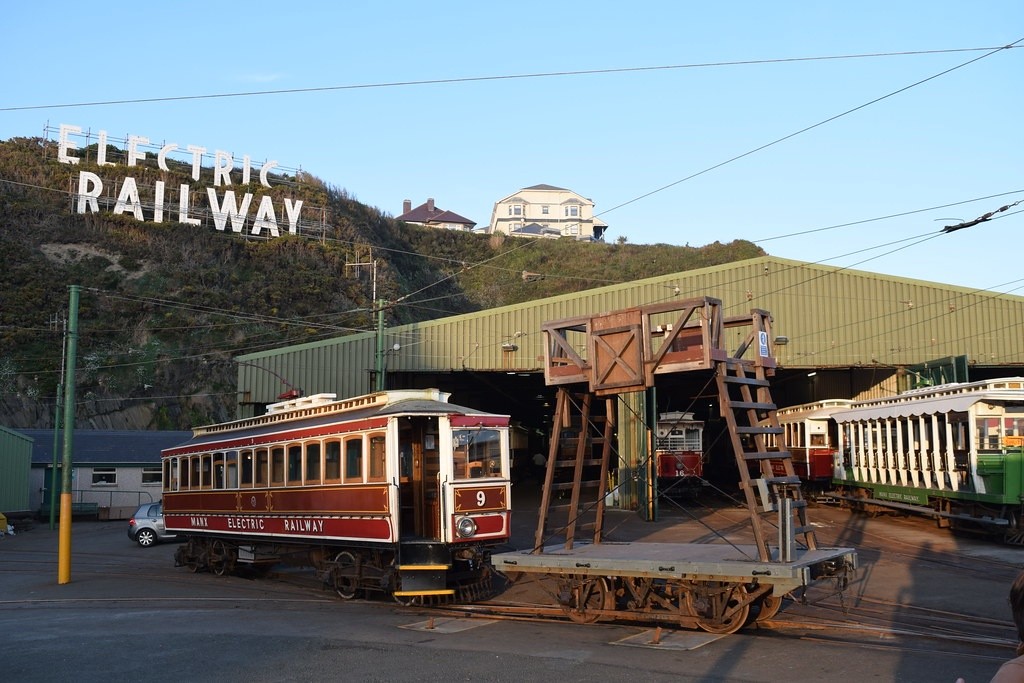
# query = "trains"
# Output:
<box><xmin>711</xmin><ymin>399</ymin><xmax>857</xmax><ymax>501</ymax></box>
<box><xmin>648</xmin><ymin>410</ymin><xmax>710</xmax><ymax>501</ymax></box>
<box><xmin>828</xmin><ymin>378</ymin><xmax>1024</xmax><ymax>546</ymax></box>
<box><xmin>160</xmin><ymin>388</ymin><xmax>513</xmax><ymax>608</ymax></box>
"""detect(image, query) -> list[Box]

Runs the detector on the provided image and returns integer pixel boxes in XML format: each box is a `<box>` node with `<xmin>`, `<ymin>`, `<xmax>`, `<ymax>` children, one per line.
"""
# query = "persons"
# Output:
<box><xmin>956</xmin><ymin>572</ymin><xmax>1024</xmax><ymax>683</ymax></box>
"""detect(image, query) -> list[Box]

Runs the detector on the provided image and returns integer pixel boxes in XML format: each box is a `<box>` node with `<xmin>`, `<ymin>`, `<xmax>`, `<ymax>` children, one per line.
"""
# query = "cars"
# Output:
<box><xmin>128</xmin><ymin>501</ymin><xmax>177</xmax><ymax>548</ymax></box>
